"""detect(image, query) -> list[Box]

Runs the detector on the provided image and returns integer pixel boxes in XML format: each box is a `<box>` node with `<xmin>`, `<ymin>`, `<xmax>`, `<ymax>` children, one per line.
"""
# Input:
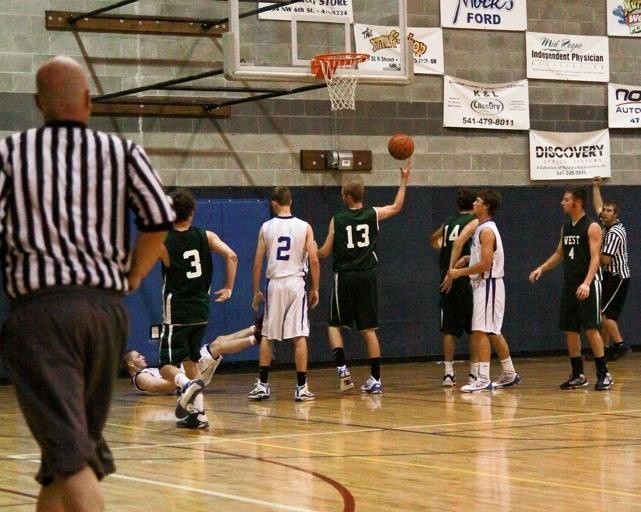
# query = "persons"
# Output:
<box><xmin>249</xmin><ymin>186</ymin><xmax>317</xmax><ymax>403</ymax></box>
<box><xmin>0</xmin><ymin>59</ymin><xmax>175</xmax><ymax>512</ymax></box>
<box><xmin>135</xmin><ymin>187</ymin><xmax>238</xmax><ymax>432</ymax></box>
<box><xmin>529</xmin><ymin>187</ymin><xmax>614</xmax><ymax>389</ymax></box>
<box><xmin>121</xmin><ymin>310</ymin><xmax>267</xmax><ymax>397</ymax></box>
<box><xmin>315</xmin><ymin>162</ymin><xmax>417</xmax><ymax>396</ymax></box>
<box><xmin>449</xmin><ymin>191</ymin><xmax>522</xmax><ymax>389</ymax></box>
<box><xmin>583</xmin><ymin>175</ymin><xmax>632</xmax><ymax>362</ymax></box>
<box><xmin>430</xmin><ymin>189</ymin><xmax>483</xmax><ymax>389</ymax></box>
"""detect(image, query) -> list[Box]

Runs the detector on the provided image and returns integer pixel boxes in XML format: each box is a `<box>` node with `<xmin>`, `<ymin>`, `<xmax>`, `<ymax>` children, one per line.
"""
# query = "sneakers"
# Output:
<box><xmin>175</xmin><ymin>408</ymin><xmax>209</xmax><ymax>429</ymax></box>
<box><xmin>586</xmin><ymin>342</ymin><xmax>633</xmax><ymax>362</ymax></box>
<box><xmin>594</xmin><ymin>371</ymin><xmax>616</xmax><ymax>391</ymax></box>
<box><xmin>559</xmin><ymin>371</ymin><xmax>589</xmax><ymax>390</ymax></box>
<box><xmin>336</xmin><ymin>363</ymin><xmax>355</xmax><ymax>393</ymax></box>
<box><xmin>491</xmin><ymin>373</ymin><xmax>522</xmax><ymax>390</ymax></box>
<box><xmin>247</xmin><ymin>379</ymin><xmax>271</xmax><ymax>400</ymax></box>
<box><xmin>360</xmin><ymin>375</ymin><xmax>383</xmax><ymax>395</ymax></box>
<box><xmin>294</xmin><ymin>377</ymin><xmax>316</xmax><ymax>402</ymax></box>
<box><xmin>441</xmin><ymin>371</ymin><xmax>457</xmax><ymax>387</ymax></box>
<box><xmin>459</xmin><ymin>373</ymin><xmax>494</xmax><ymax>393</ymax></box>
<box><xmin>175</xmin><ymin>378</ymin><xmax>205</xmax><ymax>420</ymax></box>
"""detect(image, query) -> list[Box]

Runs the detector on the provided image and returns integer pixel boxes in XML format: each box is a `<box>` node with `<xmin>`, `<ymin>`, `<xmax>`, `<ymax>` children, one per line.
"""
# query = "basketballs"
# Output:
<box><xmin>388</xmin><ymin>133</ymin><xmax>414</xmax><ymax>159</ymax></box>
